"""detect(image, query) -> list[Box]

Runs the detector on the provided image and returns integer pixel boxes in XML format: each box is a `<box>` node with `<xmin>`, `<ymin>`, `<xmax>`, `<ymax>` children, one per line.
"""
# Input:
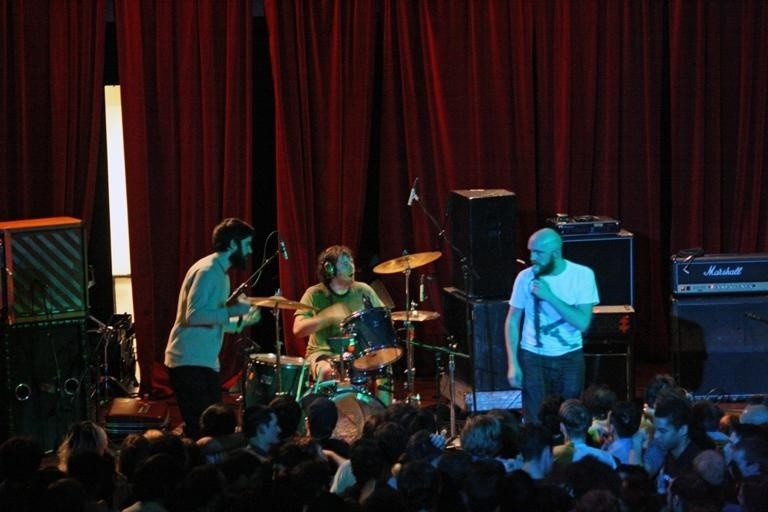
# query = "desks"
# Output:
<box><xmin>582</xmin><ymin>305</ymin><xmax>635</xmax><ymax>401</ymax></box>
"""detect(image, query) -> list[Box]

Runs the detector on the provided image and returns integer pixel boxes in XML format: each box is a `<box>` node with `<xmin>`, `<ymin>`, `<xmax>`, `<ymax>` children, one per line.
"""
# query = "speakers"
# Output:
<box><xmin>0</xmin><ymin>325</ymin><xmax>96</xmax><ymax>457</ymax></box>
<box><xmin>442</xmin><ymin>285</ymin><xmax>523</xmax><ymax>390</ymax></box>
<box><xmin>670</xmin><ymin>294</ymin><xmax>768</xmax><ymax>404</ymax></box>
<box><xmin>446</xmin><ymin>187</ymin><xmax>517</xmax><ymax>300</ymax></box>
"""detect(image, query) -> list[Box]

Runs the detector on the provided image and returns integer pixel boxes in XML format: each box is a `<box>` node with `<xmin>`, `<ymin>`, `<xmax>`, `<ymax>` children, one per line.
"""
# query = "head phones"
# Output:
<box><xmin>322</xmin><ymin>247</ymin><xmax>337</xmax><ymax>276</ymax></box>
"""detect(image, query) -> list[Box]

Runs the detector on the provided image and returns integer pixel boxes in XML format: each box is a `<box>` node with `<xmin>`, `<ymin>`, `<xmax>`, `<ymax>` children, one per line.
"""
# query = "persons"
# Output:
<box><xmin>503</xmin><ymin>227</ymin><xmax>600</xmax><ymax>426</ymax></box>
<box><xmin>1</xmin><ymin>393</ymin><xmax>766</xmax><ymax>511</ymax></box>
<box><xmin>160</xmin><ymin>215</ymin><xmax>257</xmax><ymax>436</ymax></box>
<box><xmin>292</xmin><ymin>244</ymin><xmax>394</xmax><ymax>407</ymax></box>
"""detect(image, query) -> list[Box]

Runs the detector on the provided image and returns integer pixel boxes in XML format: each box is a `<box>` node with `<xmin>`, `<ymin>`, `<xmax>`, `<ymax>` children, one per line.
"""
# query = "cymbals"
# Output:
<box><xmin>373</xmin><ymin>252</ymin><xmax>442</xmax><ymax>274</ymax></box>
<box><xmin>238</xmin><ymin>295</ymin><xmax>319</xmax><ymax>313</ymax></box>
<box><xmin>391</xmin><ymin>310</ymin><xmax>440</xmax><ymax>322</ymax></box>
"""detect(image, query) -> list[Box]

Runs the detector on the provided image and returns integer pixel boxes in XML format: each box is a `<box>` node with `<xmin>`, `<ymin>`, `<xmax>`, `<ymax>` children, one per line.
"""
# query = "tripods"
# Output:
<box><xmin>91</xmin><ymin>330</ymin><xmax>128</xmax><ymax>404</ymax></box>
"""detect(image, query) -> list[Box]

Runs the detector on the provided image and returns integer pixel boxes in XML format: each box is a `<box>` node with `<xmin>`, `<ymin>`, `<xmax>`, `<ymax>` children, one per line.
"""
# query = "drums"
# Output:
<box><xmin>299</xmin><ymin>380</ymin><xmax>387</xmax><ymax>446</ymax></box>
<box><xmin>341</xmin><ymin>307</ymin><xmax>405</xmax><ymax>374</ymax></box>
<box><xmin>245</xmin><ymin>353</ymin><xmax>310</xmax><ymax>410</ymax></box>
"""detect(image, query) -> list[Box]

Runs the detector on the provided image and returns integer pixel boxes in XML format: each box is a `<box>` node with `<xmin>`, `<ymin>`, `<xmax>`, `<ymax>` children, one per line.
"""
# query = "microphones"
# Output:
<box><xmin>415</xmin><ymin>272</ymin><xmax>427</xmax><ymax>302</ymax></box>
<box><xmin>531</xmin><ymin>263</ymin><xmax>543</xmax><ymax>303</ymax></box>
<box><xmin>742</xmin><ymin>309</ymin><xmax>767</xmax><ymax>324</ymax></box>
<box><xmin>407</xmin><ymin>174</ymin><xmax>420</xmax><ymax>207</ymax></box>
<box><xmin>280</xmin><ymin>238</ymin><xmax>289</xmax><ymax>260</ymax></box>
